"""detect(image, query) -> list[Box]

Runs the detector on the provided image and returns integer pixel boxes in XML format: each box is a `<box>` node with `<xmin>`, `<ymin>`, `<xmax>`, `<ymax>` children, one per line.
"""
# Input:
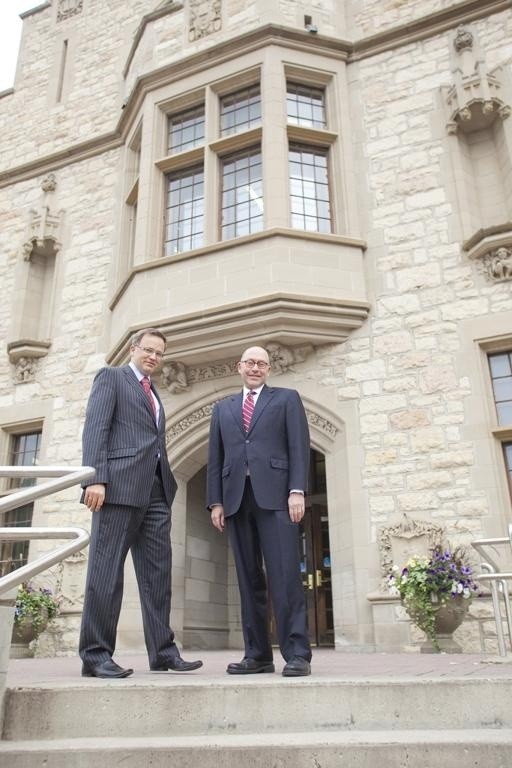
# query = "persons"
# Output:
<box><xmin>80</xmin><ymin>327</ymin><xmax>202</xmax><ymax>677</ymax></box>
<box><xmin>207</xmin><ymin>346</ymin><xmax>312</xmax><ymax>677</ymax></box>
<box><xmin>17</xmin><ymin>357</ymin><xmax>32</xmax><ymax>381</ymax></box>
<box><xmin>491</xmin><ymin>246</ymin><xmax>512</xmax><ymax>277</ymax></box>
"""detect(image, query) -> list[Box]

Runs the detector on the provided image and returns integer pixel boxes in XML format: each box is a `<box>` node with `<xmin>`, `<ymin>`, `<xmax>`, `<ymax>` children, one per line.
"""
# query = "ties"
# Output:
<box><xmin>141</xmin><ymin>377</ymin><xmax>157</xmax><ymax>418</ymax></box>
<box><xmin>242</xmin><ymin>391</ymin><xmax>255</xmax><ymax>433</ymax></box>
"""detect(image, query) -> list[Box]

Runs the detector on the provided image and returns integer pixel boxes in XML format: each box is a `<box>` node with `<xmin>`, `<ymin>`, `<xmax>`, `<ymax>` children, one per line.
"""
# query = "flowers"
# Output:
<box><xmin>14</xmin><ymin>580</ymin><xmax>75</xmax><ymax>657</ymax></box>
<box><xmin>387</xmin><ymin>540</ymin><xmax>485</xmax><ymax>651</ymax></box>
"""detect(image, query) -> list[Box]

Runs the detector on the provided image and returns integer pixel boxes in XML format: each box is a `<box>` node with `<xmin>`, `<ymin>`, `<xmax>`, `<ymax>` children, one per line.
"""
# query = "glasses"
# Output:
<box><xmin>243</xmin><ymin>358</ymin><xmax>271</xmax><ymax>370</ymax></box>
<box><xmin>135</xmin><ymin>344</ymin><xmax>163</xmax><ymax>359</ymax></box>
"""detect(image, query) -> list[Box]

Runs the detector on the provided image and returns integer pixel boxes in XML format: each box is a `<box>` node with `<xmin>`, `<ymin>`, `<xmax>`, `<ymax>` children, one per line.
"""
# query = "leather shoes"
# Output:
<box><xmin>82</xmin><ymin>658</ymin><xmax>133</xmax><ymax>679</ymax></box>
<box><xmin>226</xmin><ymin>657</ymin><xmax>275</xmax><ymax>674</ymax></box>
<box><xmin>282</xmin><ymin>656</ymin><xmax>311</xmax><ymax>676</ymax></box>
<box><xmin>149</xmin><ymin>655</ymin><xmax>203</xmax><ymax>672</ymax></box>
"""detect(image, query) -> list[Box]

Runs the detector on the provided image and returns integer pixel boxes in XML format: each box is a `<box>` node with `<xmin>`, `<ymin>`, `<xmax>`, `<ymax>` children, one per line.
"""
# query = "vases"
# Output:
<box><xmin>9</xmin><ymin>606</ymin><xmax>50</xmax><ymax>660</ymax></box>
<box><xmin>401</xmin><ymin>590</ymin><xmax>481</xmax><ymax>653</ymax></box>
